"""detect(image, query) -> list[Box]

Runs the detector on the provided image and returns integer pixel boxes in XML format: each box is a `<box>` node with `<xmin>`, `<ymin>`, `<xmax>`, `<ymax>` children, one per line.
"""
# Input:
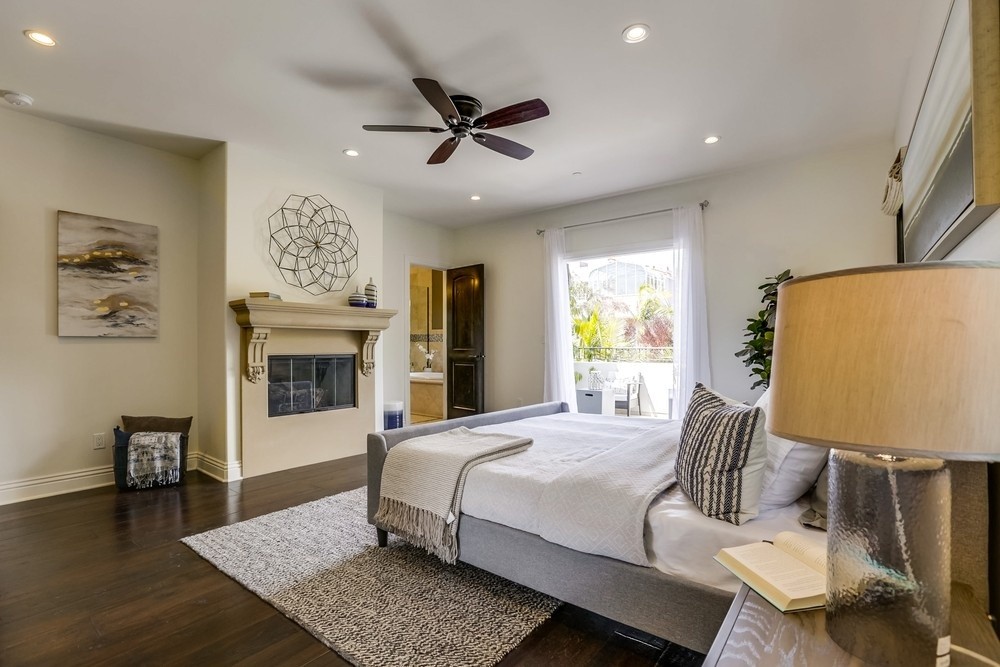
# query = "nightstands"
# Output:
<box><xmin>701</xmin><ymin>538</ymin><xmax>999</xmax><ymax>667</ymax></box>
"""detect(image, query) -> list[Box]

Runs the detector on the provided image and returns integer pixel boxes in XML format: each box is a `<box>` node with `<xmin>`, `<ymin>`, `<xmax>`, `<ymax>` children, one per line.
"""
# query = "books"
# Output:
<box><xmin>713</xmin><ymin>530</ymin><xmax>916</xmax><ymax>614</ymax></box>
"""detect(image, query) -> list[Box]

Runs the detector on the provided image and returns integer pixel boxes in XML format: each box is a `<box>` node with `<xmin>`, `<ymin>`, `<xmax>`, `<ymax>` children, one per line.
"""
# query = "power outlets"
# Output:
<box><xmin>92</xmin><ymin>433</ymin><xmax>106</xmax><ymax>449</ymax></box>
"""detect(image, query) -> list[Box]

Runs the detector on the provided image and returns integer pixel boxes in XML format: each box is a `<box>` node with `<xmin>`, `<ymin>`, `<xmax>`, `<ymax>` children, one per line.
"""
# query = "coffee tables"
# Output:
<box><xmin>577</xmin><ymin>388</ymin><xmax>615</xmax><ymax>414</ymax></box>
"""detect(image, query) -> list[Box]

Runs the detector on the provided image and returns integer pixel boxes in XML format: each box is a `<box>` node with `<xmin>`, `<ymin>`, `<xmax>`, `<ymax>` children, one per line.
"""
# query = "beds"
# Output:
<box><xmin>367</xmin><ymin>398</ymin><xmax>990</xmax><ymax>654</ymax></box>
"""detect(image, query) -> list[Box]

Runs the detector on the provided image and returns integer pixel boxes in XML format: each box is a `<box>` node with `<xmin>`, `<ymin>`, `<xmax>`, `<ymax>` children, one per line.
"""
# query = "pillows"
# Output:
<box><xmin>122</xmin><ymin>413</ymin><xmax>194</xmax><ymax>435</ymax></box>
<box><xmin>751</xmin><ymin>386</ymin><xmax>829</xmax><ymax>511</ymax></box>
<box><xmin>674</xmin><ymin>381</ymin><xmax>764</xmax><ymax>526</ymax></box>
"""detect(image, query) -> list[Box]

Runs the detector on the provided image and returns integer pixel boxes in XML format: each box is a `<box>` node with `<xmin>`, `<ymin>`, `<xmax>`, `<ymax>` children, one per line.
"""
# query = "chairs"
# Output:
<box><xmin>616</xmin><ymin>373</ymin><xmax>641</xmax><ymax>417</ymax></box>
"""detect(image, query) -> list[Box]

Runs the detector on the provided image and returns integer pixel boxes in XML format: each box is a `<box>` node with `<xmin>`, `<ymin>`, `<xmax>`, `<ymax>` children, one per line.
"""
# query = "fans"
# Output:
<box><xmin>362</xmin><ymin>78</ymin><xmax>550</xmax><ymax>164</ymax></box>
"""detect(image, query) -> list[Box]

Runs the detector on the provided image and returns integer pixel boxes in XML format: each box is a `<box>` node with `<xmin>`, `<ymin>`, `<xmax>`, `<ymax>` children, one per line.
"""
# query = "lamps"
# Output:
<box><xmin>764</xmin><ymin>261</ymin><xmax>999</xmax><ymax>667</ymax></box>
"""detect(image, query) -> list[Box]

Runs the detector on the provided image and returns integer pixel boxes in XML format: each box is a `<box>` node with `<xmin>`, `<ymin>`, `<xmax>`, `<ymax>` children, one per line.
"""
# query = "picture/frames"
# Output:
<box><xmin>896</xmin><ymin>0</ymin><xmax>1000</xmax><ymax>262</ymax></box>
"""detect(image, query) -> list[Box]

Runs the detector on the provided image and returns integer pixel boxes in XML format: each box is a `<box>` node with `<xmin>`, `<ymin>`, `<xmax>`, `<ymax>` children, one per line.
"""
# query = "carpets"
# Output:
<box><xmin>177</xmin><ymin>485</ymin><xmax>564</xmax><ymax>667</ymax></box>
